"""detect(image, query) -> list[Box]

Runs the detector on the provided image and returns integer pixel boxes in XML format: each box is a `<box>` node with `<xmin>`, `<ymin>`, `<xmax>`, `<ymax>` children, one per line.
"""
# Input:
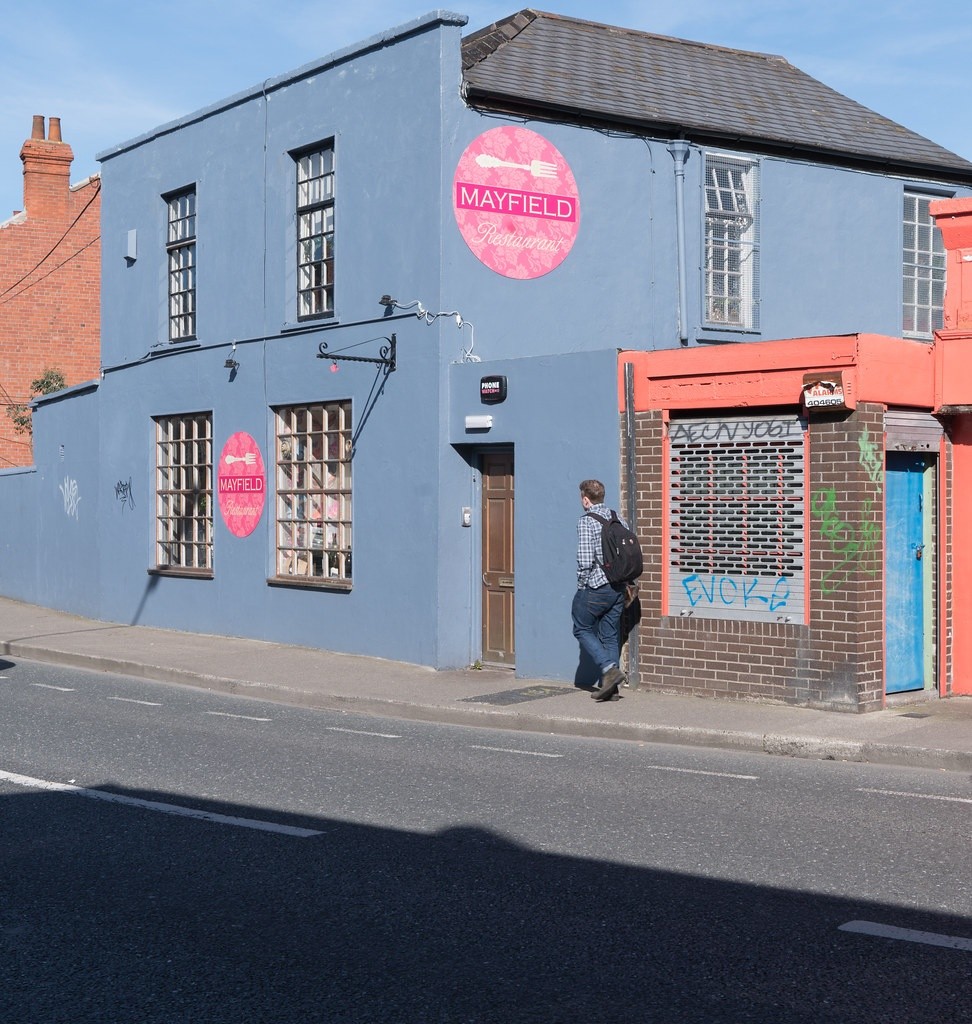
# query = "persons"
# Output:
<box><xmin>571</xmin><ymin>479</ymin><xmax>644</xmax><ymax>702</ymax></box>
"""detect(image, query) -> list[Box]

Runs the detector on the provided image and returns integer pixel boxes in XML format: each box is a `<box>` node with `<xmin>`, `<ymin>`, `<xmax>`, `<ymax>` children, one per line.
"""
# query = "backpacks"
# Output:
<box><xmin>582</xmin><ymin>509</ymin><xmax>644</xmax><ymax>584</ymax></box>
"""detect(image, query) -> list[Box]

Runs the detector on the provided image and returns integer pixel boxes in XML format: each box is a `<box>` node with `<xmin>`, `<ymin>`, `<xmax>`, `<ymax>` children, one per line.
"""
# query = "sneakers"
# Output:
<box><xmin>590</xmin><ymin>687</ymin><xmax>619</xmax><ymax>701</ymax></box>
<box><xmin>595</xmin><ymin>667</ymin><xmax>625</xmax><ymax>699</ymax></box>
